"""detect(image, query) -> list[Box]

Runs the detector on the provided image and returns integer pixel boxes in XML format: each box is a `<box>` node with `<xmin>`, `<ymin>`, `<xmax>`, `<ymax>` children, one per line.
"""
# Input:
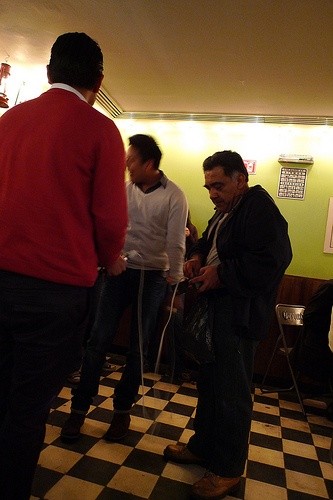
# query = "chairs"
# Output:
<box><xmin>261</xmin><ymin>304</ymin><xmax>309</xmax><ymax>417</ymax></box>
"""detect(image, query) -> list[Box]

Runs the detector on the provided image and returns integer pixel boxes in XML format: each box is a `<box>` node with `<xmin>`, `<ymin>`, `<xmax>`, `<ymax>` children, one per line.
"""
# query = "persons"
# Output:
<box><xmin>163</xmin><ymin>151</ymin><xmax>293</xmax><ymax>500</ymax></box>
<box><xmin>58</xmin><ymin>135</ymin><xmax>188</xmax><ymax>444</ymax></box>
<box><xmin>294</xmin><ymin>279</ymin><xmax>333</xmax><ymax>422</ymax></box>
<box><xmin>0</xmin><ymin>32</ymin><xmax>128</xmax><ymax>500</ymax></box>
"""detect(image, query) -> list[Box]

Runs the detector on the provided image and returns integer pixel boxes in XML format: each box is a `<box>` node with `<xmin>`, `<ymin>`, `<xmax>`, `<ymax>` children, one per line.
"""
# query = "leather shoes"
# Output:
<box><xmin>191</xmin><ymin>470</ymin><xmax>240</xmax><ymax>500</ymax></box>
<box><xmin>164</xmin><ymin>445</ymin><xmax>210</xmax><ymax>465</ymax></box>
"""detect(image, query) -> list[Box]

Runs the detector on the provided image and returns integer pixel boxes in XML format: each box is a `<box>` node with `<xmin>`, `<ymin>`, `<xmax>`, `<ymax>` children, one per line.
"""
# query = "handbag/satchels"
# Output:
<box><xmin>181</xmin><ymin>296</ymin><xmax>216</xmax><ymax>366</ymax></box>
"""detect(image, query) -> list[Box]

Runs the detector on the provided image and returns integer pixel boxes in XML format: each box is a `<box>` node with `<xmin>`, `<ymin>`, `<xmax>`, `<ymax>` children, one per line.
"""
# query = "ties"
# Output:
<box><xmin>206</xmin><ymin>213</ymin><xmax>224</xmax><ymax>257</ymax></box>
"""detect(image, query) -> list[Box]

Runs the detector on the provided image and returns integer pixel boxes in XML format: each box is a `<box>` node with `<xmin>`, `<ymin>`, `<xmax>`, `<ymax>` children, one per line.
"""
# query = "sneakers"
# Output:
<box><xmin>106</xmin><ymin>415</ymin><xmax>130</xmax><ymax>440</ymax></box>
<box><xmin>61</xmin><ymin>413</ymin><xmax>85</xmax><ymax>442</ymax></box>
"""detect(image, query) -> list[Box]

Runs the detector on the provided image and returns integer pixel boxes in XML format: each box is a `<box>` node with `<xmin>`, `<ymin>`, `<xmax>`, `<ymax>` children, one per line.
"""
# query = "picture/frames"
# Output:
<box><xmin>323</xmin><ymin>197</ymin><xmax>333</xmax><ymax>253</ymax></box>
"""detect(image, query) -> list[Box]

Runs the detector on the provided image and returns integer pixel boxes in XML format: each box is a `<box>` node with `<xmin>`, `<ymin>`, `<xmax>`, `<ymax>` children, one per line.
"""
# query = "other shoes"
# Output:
<box><xmin>66</xmin><ymin>370</ymin><xmax>81</xmax><ymax>383</ymax></box>
<box><xmin>327</xmin><ymin>404</ymin><xmax>333</xmax><ymax>420</ymax></box>
<box><xmin>167</xmin><ymin>368</ymin><xmax>181</xmax><ymax>384</ymax></box>
<box><xmin>192</xmin><ymin>371</ymin><xmax>200</xmax><ymax>381</ymax></box>
<box><xmin>100</xmin><ymin>368</ymin><xmax>108</xmax><ymax>376</ymax></box>
